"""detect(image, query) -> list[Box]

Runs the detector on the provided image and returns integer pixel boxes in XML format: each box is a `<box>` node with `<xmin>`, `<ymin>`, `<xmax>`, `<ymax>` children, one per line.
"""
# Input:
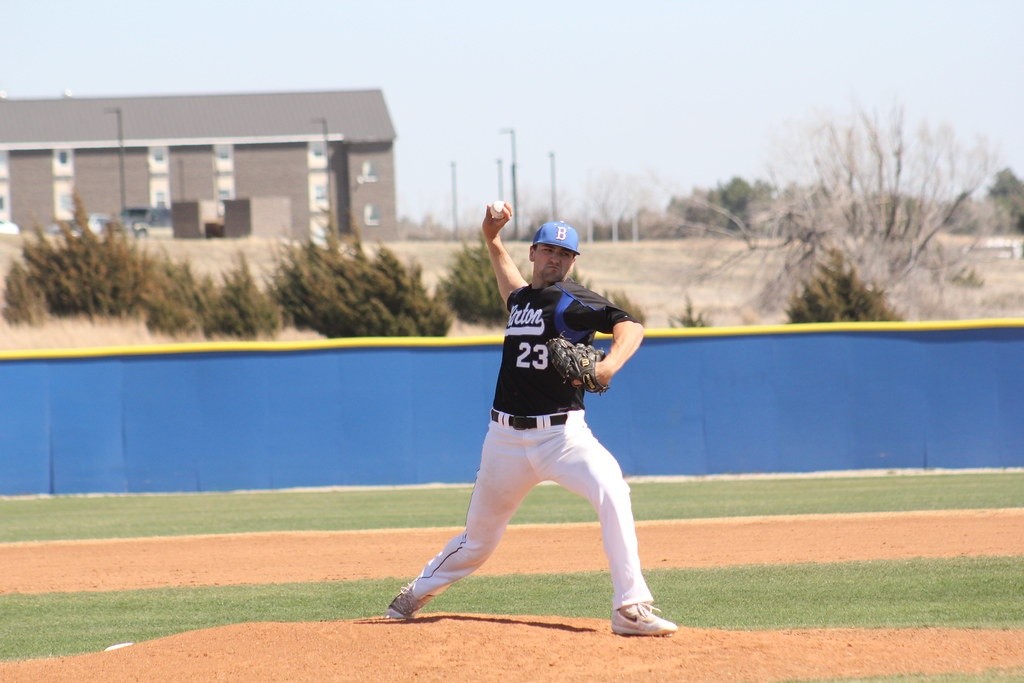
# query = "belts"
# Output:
<box><xmin>491</xmin><ymin>409</ymin><xmax>568</xmax><ymax>431</ymax></box>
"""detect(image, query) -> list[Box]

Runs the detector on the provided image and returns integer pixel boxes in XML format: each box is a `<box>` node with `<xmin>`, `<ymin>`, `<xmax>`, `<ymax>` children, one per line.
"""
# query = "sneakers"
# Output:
<box><xmin>611</xmin><ymin>603</ymin><xmax>678</xmax><ymax>635</ymax></box>
<box><xmin>388</xmin><ymin>582</ymin><xmax>426</xmax><ymax>619</ymax></box>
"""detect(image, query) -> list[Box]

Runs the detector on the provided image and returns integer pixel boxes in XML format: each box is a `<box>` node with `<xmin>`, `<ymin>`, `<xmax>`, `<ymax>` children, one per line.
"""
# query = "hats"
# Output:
<box><xmin>530</xmin><ymin>219</ymin><xmax>580</xmax><ymax>256</ymax></box>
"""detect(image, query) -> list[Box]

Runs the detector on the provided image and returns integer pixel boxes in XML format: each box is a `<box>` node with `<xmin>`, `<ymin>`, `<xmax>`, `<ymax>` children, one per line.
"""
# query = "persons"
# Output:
<box><xmin>388</xmin><ymin>202</ymin><xmax>677</xmax><ymax>638</ymax></box>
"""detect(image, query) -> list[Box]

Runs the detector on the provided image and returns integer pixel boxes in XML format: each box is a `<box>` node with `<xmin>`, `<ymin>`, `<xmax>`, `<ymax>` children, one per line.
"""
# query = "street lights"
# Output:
<box><xmin>500</xmin><ymin>127</ymin><xmax>518</xmax><ymax>240</ymax></box>
<box><xmin>104</xmin><ymin>107</ymin><xmax>125</xmax><ymax>232</ymax></box>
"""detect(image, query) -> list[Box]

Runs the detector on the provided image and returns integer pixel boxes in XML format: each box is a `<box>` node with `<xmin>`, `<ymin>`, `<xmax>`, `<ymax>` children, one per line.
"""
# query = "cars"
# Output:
<box><xmin>64</xmin><ymin>214</ymin><xmax>112</xmax><ymax>239</ymax></box>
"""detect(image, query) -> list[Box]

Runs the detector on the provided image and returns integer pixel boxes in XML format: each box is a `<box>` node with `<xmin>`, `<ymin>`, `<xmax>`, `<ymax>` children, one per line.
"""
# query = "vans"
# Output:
<box><xmin>121</xmin><ymin>206</ymin><xmax>174</xmax><ymax>238</ymax></box>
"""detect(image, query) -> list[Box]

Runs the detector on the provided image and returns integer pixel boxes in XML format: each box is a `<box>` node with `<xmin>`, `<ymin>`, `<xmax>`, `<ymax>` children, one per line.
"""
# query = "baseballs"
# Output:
<box><xmin>490</xmin><ymin>201</ymin><xmax>505</xmax><ymax>219</ymax></box>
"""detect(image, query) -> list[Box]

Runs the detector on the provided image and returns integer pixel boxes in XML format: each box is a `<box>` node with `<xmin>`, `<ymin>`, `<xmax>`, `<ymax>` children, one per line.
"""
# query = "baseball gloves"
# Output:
<box><xmin>546</xmin><ymin>336</ymin><xmax>611</xmax><ymax>394</ymax></box>
